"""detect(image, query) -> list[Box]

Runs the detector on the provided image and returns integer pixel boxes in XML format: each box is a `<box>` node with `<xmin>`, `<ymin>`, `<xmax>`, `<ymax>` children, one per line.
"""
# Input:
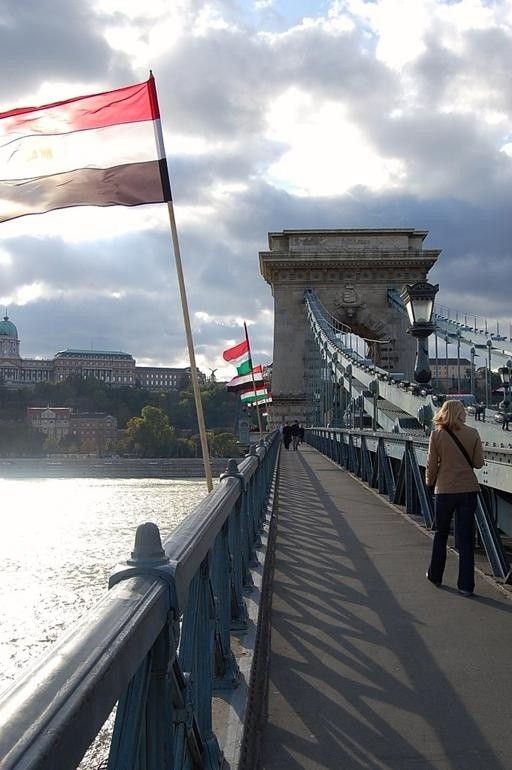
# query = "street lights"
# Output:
<box><xmin>400</xmin><ymin>282</ymin><xmax>440</xmax><ymax>387</ymax></box>
<box><xmin>498</xmin><ymin>366</ymin><xmax>510</xmax><ymax>400</ymax></box>
<box><xmin>433</xmin><ymin>325</ymin><xmax>492</xmax><ymax>409</ymax></box>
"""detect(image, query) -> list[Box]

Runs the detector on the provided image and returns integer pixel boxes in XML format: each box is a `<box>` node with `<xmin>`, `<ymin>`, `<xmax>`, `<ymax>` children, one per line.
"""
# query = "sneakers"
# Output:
<box><xmin>458</xmin><ymin>589</ymin><xmax>472</xmax><ymax>596</ymax></box>
<box><xmin>426</xmin><ymin>572</ymin><xmax>442</xmax><ymax>587</ymax></box>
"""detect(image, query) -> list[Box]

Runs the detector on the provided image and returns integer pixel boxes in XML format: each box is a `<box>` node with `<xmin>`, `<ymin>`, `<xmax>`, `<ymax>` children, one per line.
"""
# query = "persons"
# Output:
<box><xmin>472</xmin><ymin>399</ymin><xmax>511</xmax><ymax>430</ymax></box>
<box><xmin>425</xmin><ymin>399</ymin><xmax>486</xmax><ymax>597</ymax></box>
<box><xmin>278</xmin><ymin>417</ymin><xmax>311</xmax><ymax>452</ymax></box>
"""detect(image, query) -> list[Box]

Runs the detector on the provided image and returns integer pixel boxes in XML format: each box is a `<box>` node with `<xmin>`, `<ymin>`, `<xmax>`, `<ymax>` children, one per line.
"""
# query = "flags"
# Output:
<box><xmin>222</xmin><ymin>340</ymin><xmax>252</xmax><ymax>376</ymax></box>
<box><xmin>247</xmin><ymin>391</ymin><xmax>272</xmax><ymax>409</ymax></box>
<box><xmin>251</xmin><ymin>408</ymin><xmax>270</xmax><ymax>426</ymax></box>
<box><xmin>0</xmin><ymin>78</ymin><xmax>173</xmax><ymax>223</ymax></box>
<box><xmin>226</xmin><ymin>364</ymin><xmax>265</xmax><ymax>392</ymax></box>
<box><xmin>240</xmin><ymin>383</ymin><xmax>269</xmax><ymax>403</ymax></box>
